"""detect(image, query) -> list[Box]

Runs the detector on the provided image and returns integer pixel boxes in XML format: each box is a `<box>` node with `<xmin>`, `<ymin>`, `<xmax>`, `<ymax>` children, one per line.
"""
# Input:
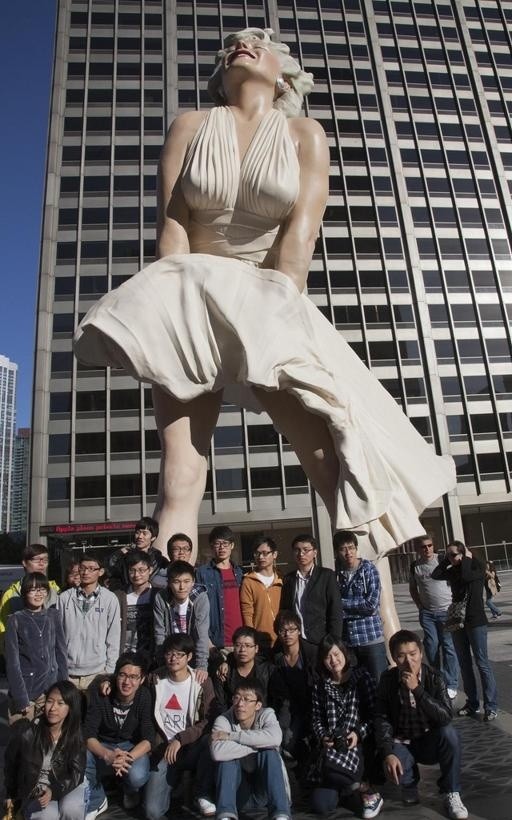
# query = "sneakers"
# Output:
<box><xmin>402</xmin><ymin>789</ymin><xmax>421</xmax><ymax>804</ymax></box>
<box><xmin>84</xmin><ymin>798</ymin><xmax>109</xmax><ymax>818</ymax></box>
<box><xmin>484</xmin><ymin>709</ymin><xmax>497</xmax><ymax>722</ymax></box>
<box><xmin>195</xmin><ymin>797</ymin><xmax>216</xmax><ymax>814</ymax></box>
<box><xmin>362</xmin><ymin>792</ymin><xmax>383</xmax><ymax>819</ymax></box>
<box><xmin>459</xmin><ymin>702</ymin><xmax>480</xmax><ymax>715</ymax></box>
<box><xmin>447</xmin><ymin>687</ymin><xmax>457</xmax><ymax>699</ymax></box>
<box><xmin>444</xmin><ymin>792</ymin><xmax>468</xmax><ymax>819</ymax></box>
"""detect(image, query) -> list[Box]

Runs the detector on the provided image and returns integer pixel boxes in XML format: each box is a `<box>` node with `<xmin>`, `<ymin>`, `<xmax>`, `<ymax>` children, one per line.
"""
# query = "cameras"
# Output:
<box><xmin>333</xmin><ymin>730</ymin><xmax>349</xmax><ymax>751</ymax></box>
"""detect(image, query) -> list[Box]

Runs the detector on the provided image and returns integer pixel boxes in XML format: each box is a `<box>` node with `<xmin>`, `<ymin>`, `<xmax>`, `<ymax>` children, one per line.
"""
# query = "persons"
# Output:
<box><xmin>114</xmin><ymin>551</ymin><xmax>159</xmax><ymax>687</ymax></box>
<box><xmin>431</xmin><ymin>541</ymin><xmax>497</xmax><ymax>723</ymax></box>
<box><xmin>485</xmin><ymin>560</ymin><xmax>502</xmax><ymax>621</ymax></box>
<box><xmin>0</xmin><ymin>544</ymin><xmax>62</xmax><ymax>663</ymax></box>
<box><xmin>4</xmin><ymin>681</ymin><xmax>87</xmax><ymax>820</ymax></box>
<box><xmin>59</xmin><ymin>562</ymin><xmax>81</xmax><ymax>595</ymax></box>
<box><xmin>194</xmin><ymin>526</ymin><xmax>245</xmax><ymax>660</ymax></box>
<box><xmin>283</xmin><ymin>535</ymin><xmax>344</xmax><ymax>679</ymax></box>
<box><xmin>72</xmin><ymin>26</ymin><xmax>456</xmax><ymax>668</ymax></box>
<box><xmin>239</xmin><ymin>538</ymin><xmax>285</xmax><ymax>653</ymax></box>
<box><xmin>151</xmin><ymin>533</ymin><xmax>192</xmax><ymax>588</ymax></box>
<box><xmin>409</xmin><ymin>536</ymin><xmax>458</xmax><ymax>699</ymax></box>
<box><xmin>208</xmin><ymin>678</ymin><xmax>291</xmax><ymax>820</ymax></box>
<box><xmin>4</xmin><ymin>572</ymin><xmax>68</xmax><ymax>725</ymax></box>
<box><xmin>374</xmin><ymin>629</ymin><xmax>469</xmax><ymax>819</ymax></box>
<box><xmin>101</xmin><ymin>567</ymin><xmax>127</xmax><ymax>656</ymax></box>
<box><xmin>216</xmin><ymin>626</ymin><xmax>278</xmax><ymax>715</ymax></box>
<box><xmin>109</xmin><ymin>517</ymin><xmax>169</xmax><ymax>589</ymax></box>
<box><xmin>310</xmin><ymin>634</ymin><xmax>383</xmax><ymax>819</ymax></box>
<box><xmin>154</xmin><ymin>560</ymin><xmax>210</xmax><ymax>685</ymax></box>
<box><xmin>57</xmin><ymin>552</ymin><xmax>121</xmax><ymax>702</ymax></box>
<box><xmin>217</xmin><ymin>611</ymin><xmax>320</xmax><ymax>772</ymax></box>
<box><xmin>99</xmin><ymin>633</ymin><xmax>217</xmax><ymax>816</ymax></box>
<box><xmin>82</xmin><ymin>651</ymin><xmax>157</xmax><ymax>820</ymax></box>
<box><xmin>335</xmin><ymin>531</ymin><xmax>386</xmax><ymax>687</ymax></box>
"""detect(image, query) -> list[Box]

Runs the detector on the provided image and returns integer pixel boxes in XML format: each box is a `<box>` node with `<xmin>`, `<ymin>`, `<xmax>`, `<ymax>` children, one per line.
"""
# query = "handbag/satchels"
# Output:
<box><xmin>442</xmin><ymin>596</ymin><xmax>470</xmax><ymax>633</ymax></box>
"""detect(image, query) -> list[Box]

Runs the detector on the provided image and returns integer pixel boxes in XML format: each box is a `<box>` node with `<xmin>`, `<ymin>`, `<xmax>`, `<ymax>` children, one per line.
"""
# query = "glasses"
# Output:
<box><xmin>235</xmin><ymin>642</ymin><xmax>258</xmax><ymax>648</ymax></box>
<box><xmin>231</xmin><ymin>697</ymin><xmax>259</xmax><ymax>704</ymax></box>
<box><xmin>164</xmin><ymin>653</ymin><xmax>186</xmax><ymax>659</ymax></box>
<box><xmin>170</xmin><ymin>545</ymin><xmax>190</xmax><ymax>551</ymax></box>
<box><xmin>26</xmin><ymin>556</ymin><xmax>49</xmax><ymax>563</ymax></box>
<box><xmin>340</xmin><ymin>545</ymin><xmax>357</xmax><ymax>553</ymax></box>
<box><xmin>81</xmin><ymin>566</ymin><xmax>101</xmax><ymax>573</ymax></box>
<box><xmin>440</xmin><ymin>552</ymin><xmax>462</xmax><ymax>560</ymax></box>
<box><xmin>66</xmin><ymin>571</ymin><xmax>80</xmax><ymax>577</ymax></box>
<box><xmin>418</xmin><ymin>544</ymin><xmax>435</xmax><ymax>550</ymax></box>
<box><xmin>294</xmin><ymin>547</ymin><xmax>315</xmax><ymax>555</ymax></box>
<box><xmin>251</xmin><ymin>550</ymin><xmax>277</xmax><ymax>556</ymax></box>
<box><xmin>128</xmin><ymin>567</ymin><xmax>152</xmax><ymax>572</ymax></box>
<box><xmin>119</xmin><ymin>672</ymin><xmax>142</xmax><ymax>680</ymax></box>
<box><xmin>25</xmin><ymin>588</ymin><xmax>47</xmax><ymax>595</ymax></box>
<box><xmin>275</xmin><ymin>628</ymin><xmax>297</xmax><ymax>634</ymax></box>
<box><xmin>214</xmin><ymin>539</ymin><xmax>233</xmax><ymax>549</ymax></box>
